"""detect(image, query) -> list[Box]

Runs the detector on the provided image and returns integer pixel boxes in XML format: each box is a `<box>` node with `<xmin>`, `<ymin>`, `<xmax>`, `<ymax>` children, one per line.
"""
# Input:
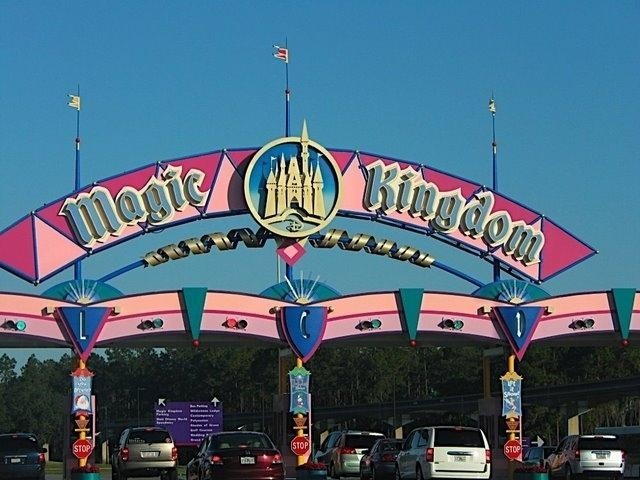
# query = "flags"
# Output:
<box><xmin>489</xmin><ymin>97</ymin><xmax>496</xmax><ymax>112</ymax></box>
<box><xmin>272</xmin><ymin>45</ymin><xmax>289</xmax><ymax>63</ymax></box>
<box><xmin>66</xmin><ymin>94</ymin><xmax>80</xmax><ymax>111</ymax></box>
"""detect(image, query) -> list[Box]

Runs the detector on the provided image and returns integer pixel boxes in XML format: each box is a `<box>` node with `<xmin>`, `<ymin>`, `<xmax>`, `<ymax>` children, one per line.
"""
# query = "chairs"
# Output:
<box><xmin>219</xmin><ymin>441</ymin><xmax>264</xmax><ymax>449</ymax></box>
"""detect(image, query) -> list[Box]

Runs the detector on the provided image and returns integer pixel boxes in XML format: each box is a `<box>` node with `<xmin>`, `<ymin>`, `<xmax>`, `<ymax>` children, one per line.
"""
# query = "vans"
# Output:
<box><xmin>544</xmin><ymin>433</ymin><xmax>626</xmax><ymax>479</ymax></box>
<box><xmin>0</xmin><ymin>433</ymin><xmax>47</xmax><ymax>479</ymax></box>
<box><xmin>394</xmin><ymin>427</ymin><xmax>491</xmax><ymax>479</ymax></box>
<box><xmin>291</xmin><ymin>436</ymin><xmax>310</xmax><ymax>456</ymax></box>
<box><xmin>315</xmin><ymin>430</ymin><xmax>386</xmax><ymax>480</ymax></box>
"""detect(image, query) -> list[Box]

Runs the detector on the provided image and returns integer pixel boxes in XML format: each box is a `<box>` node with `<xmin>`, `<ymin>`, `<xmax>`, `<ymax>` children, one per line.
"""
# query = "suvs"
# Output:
<box><xmin>73</xmin><ymin>440</ymin><xmax>92</xmax><ymax>458</ymax></box>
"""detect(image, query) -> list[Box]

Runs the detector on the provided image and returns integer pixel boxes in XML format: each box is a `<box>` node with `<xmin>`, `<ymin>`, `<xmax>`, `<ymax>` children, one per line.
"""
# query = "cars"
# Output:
<box><xmin>187</xmin><ymin>430</ymin><xmax>285</xmax><ymax>480</ymax></box>
<box><xmin>110</xmin><ymin>427</ymin><xmax>178</xmax><ymax>480</ymax></box>
<box><xmin>359</xmin><ymin>438</ymin><xmax>406</xmax><ymax>479</ymax></box>
<box><xmin>513</xmin><ymin>446</ymin><xmax>557</xmax><ymax>473</ymax></box>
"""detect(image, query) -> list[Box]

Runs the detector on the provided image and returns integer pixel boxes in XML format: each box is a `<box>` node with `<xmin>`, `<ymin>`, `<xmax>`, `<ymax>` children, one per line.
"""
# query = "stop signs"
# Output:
<box><xmin>504</xmin><ymin>439</ymin><xmax>522</xmax><ymax>460</ymax></box>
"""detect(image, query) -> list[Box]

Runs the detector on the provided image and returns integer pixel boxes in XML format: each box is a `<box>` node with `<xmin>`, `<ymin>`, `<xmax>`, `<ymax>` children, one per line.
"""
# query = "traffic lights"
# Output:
<box><xmin>572</xmin><ymin>318</ymin><xmax>594</xmax><ymax>330</ymax></box>
<box><xmin>359</xmin><ymin>319</ymin><xmax>381</xmax><ymax>330</ymax></box>
<box><xmin>141</xmin><ymin>318</ymin><xmax>162</xmax><ymax>330</ymax></box>
<box><xmin>4</xmin><ymin>319</ymin><xmax>26</xmax><ymax>331</ymax></box>
<box><xmin>444</xmin><ymin>319</ymin><xmax>463</xmax><ymax>330</ymax></box>
<box><xmin>227</xmin><ymin>318</ymin><xmax>247</xmax><ymax>330</ymax></box>
